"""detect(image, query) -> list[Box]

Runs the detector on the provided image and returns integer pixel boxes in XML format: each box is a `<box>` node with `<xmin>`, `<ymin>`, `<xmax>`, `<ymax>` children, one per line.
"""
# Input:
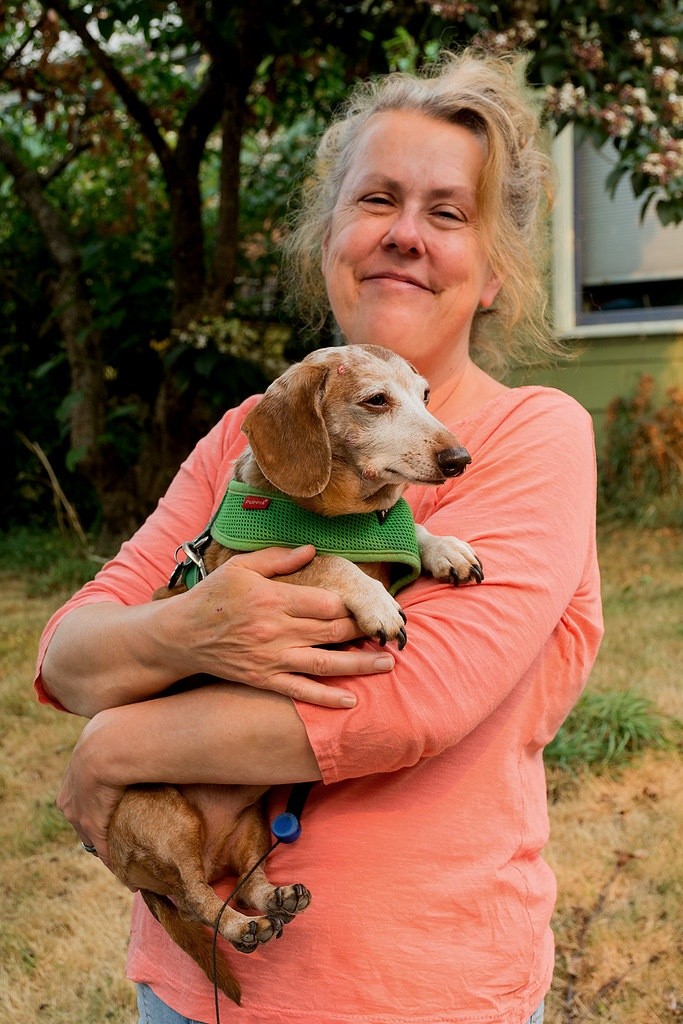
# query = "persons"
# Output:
<box><xmin>30</xmin><ymin>46</ymin><xmax>606</xmax><ymax>1024</ymax></box>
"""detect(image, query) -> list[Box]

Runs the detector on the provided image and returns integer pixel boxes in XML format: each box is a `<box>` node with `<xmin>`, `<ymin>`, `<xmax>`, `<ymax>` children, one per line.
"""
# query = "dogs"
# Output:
<box><xmin>89</xmin><ymin>344</ymin><xmax>486</xmax><ymax>1014</ymax></box>
